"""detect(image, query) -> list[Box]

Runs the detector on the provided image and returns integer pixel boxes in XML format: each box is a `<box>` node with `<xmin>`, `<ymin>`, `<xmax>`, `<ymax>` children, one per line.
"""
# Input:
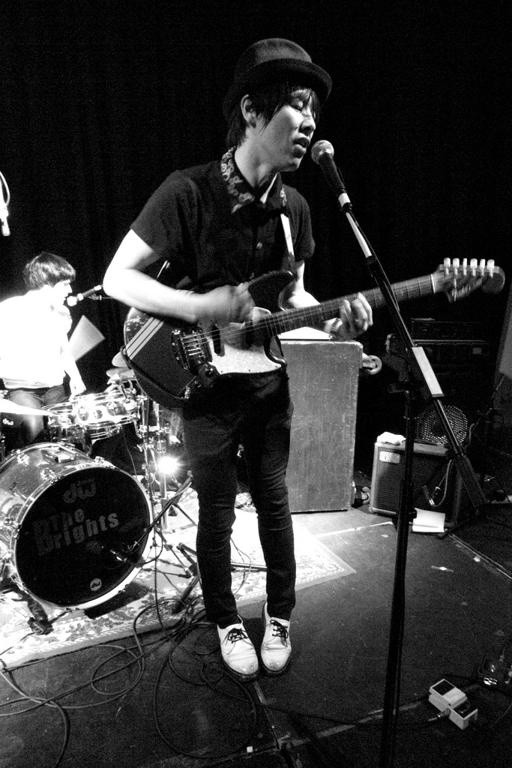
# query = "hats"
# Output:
<box><xmin>223</xmin><ymin>38</ymin><xmax>334</xmax><ymax>107</ymax></box>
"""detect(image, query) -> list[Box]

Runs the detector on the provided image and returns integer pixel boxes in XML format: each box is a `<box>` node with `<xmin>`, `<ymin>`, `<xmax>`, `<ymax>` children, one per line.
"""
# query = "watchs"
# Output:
<box><xmin>328</xmin><ymin>315</ymin><xmax>344</xmax><ymax>342</ymax></box>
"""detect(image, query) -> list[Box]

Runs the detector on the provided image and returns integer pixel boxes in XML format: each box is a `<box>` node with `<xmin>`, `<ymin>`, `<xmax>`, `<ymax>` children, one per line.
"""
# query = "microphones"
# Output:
<box><xmin>309</xmin><ymin>137</ymin><xmax>355</xmax><ymax>213</ymax></box>
<box><xmin>86</xmin><ymin>540</ymin><xmax>139</xmax><ymax>569</ymax></box>
<box><xmin>64</xmin><ymin>283</ymin><xmax>104</xmax><ymax>308</ymax></box>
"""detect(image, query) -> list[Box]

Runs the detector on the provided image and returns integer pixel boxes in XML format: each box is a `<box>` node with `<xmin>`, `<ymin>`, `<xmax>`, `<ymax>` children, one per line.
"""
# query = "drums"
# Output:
<box><xmin>1</xmin><ymin>443</ymin><xmax>154</xmax><ymax>612</ymax></box>
<box><xmin>45</xmin><ymin>387</ymin><xmax>146</xmax><ymax>468</ymax></box>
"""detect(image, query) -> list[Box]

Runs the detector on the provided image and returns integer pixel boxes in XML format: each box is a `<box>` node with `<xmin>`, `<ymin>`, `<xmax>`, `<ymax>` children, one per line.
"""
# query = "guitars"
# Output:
<box><xmin>120</xmin><ymin>256</ymin><xmax>505</xmax><ymax>410</ymax></box>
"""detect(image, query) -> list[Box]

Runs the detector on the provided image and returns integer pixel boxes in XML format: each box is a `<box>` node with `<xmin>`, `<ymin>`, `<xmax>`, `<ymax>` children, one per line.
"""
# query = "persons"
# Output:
<box><xmin>1</xmin><ymin>251</ymin><xmax>86</xmax><ymax>449</ymax></box>
<box><xmin>101</xmin><ymin>37</ymin><xmax>375</xmax><ymax>683</ymax></box>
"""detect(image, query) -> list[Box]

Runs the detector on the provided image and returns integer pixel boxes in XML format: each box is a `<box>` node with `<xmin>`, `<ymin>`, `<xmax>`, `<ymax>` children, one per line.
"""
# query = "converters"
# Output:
<box><xmin>428</xmin><ymin>680</ymin><xmax>480</xmax><ymax>728</ymax></box>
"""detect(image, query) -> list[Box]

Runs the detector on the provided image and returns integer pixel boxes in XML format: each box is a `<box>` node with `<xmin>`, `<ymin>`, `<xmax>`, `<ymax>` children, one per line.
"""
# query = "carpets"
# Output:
<box><xmin>0</xmin><ymin>474</ymin><xmax>355</xmax><ymax>670</ymax></box>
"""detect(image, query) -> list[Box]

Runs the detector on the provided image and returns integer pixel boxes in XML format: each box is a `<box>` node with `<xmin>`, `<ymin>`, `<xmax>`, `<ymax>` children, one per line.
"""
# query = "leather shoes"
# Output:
<box><xmin>217</xmin><ymin>614</ymin><xmax>259</xmax><ymax>682</ymax></box>
<box><xmin>260</xmin><ymin>601</ymin><xmax>293</xmax><ymax>675</ymax></box>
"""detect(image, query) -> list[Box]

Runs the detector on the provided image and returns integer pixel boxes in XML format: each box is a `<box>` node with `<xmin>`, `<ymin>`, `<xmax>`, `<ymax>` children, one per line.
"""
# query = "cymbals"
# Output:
<box><xmin>120</xmin><ymin>369</ymin><xmax>136</xmax><ymax>380</ymax></box>
<box><xmin>0</xmin><ymin>398</ymin><xmax>53</xmax><ymax>417</ymax></box>
<box><xmin>106</xmin><ymin>368</ymin><xmax>129</xmax><ymax>380</ymax></box>
<box><xmin>112</xmin><ymin>352</ymin><xmax>128</xmax><ymax>368</ymax></box>
